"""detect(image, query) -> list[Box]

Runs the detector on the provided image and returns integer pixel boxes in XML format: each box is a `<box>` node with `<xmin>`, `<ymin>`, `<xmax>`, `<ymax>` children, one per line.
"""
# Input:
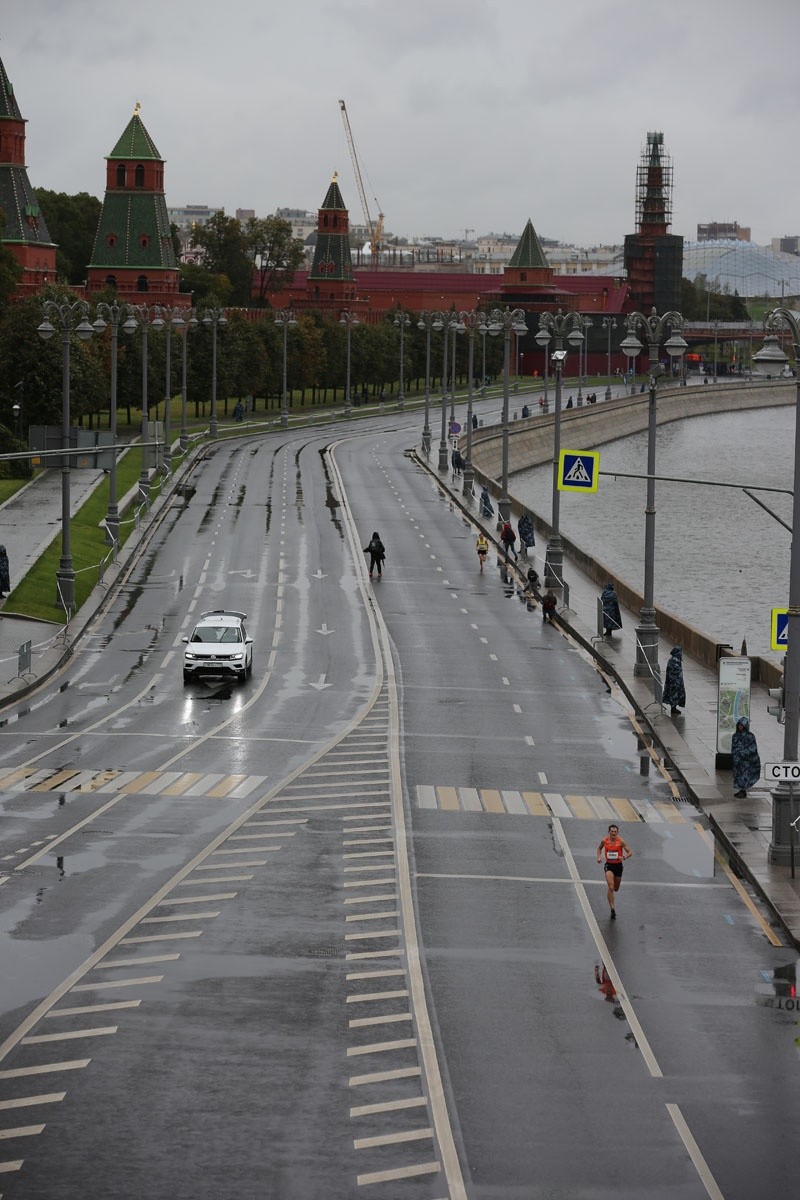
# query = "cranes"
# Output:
<box><xmin>337</xmin><ymin>98</ymin><xmax>386</xmax><ymax>264</ymax></box>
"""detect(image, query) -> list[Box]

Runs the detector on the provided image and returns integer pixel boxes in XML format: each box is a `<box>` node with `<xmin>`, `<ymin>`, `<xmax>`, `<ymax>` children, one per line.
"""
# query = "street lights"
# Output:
<box><xmin>533</xmin><ymin>310</ymin><xmax>587</xmax><ymax>591</ymax></box>
<box><xmin>35</xmin><ymin>298</ymin><xmax>96</xmax><ymax>607</ymax></box>
<box><xmin>619</xmin><ymin>309</ymin><xmax>689</xmax><ymax>678</ymax></box>
<box><xmin>91</xmin><ymin>299</ymin><xmax>529</xmax><ymax>545</ymax></box>
<box><xmin>751</xmin><ymin>308</ymin><xmax>800</xmax><ymax>871</ymax></box>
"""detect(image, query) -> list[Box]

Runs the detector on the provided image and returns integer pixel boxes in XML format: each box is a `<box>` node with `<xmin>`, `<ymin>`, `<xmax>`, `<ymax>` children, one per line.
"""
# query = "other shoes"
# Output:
<box><xmin>379</xmin><ymin>574</ymin><xmax>382</xmax><ymax>576</ymax></box>
<box><xmin>604</xmin><ymin>632</ymin><xmax>612</xmax><ymax>636</ymax></box>
<box><xmin>370</xmin><ymin>573</ymin><xmax>372</xmax><ymax>575</ymax></box>
<box><xmin>671</xmin><ymin>707</ymin><xmax>681</xmax><ymax>713</ymax></box>
<box><xmin>734</xmin><ymin>790</ymin><xmax>746</xmax><ymax>798</ymax></box>
<box><xmin>0</xmin><ymin>594</ymin><xmax>6</xmax><ymax>598</ymax></box>
<box><xmin>611</xmin><ymin>910</ymin><xmax>616</xmax><ymax>916</ymax></box>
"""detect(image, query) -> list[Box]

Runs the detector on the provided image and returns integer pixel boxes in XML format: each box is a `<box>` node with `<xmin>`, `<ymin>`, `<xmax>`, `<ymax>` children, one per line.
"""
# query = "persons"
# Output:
<box><xmin>601</xmin><ymin>582</ymin><xmax>623</xmax><ymax>637</ymax></box>
<box><xmin>476</xmin><ymin>533</ymin><xmax>488</xmax><ymax>570</ymax></box>
<box><xmin>704</xmin><ymin>377</ymin><xmax>708</xmax><ymax>384</ymax></box>
<box><xmin>521</xmin><ymin>405</ymin><xmax>528</xmax><ymax>419</ymax></box>
<box><xmin>353</xmin><ymin>391</ymin><xmax>360</xmax><ymax>407</ymax></box>
<box><xmin>363</xmin><ymin>389</ymin><xmax>369</xmax><ymax>404</ymax></box>
<box><xmin>0</xmin><ymin>544</ymin><xmax>12</xmax><ymax>600</ymax></box>
<box><xmin>540</xmin><ymin>590</ymin><xmax>557</xmax><ymax>623</ymax></box>
<box><xmin>597</xmin><ymin>825</ymin><xmax>633</xmax><ymax>918</ymax></box>
<box><xmin>472</xmin><ymin>414</ymin><xmax>477</xmax><ymax>429</ymax></box>
<box><xmin>731</xmin><ymin>717</ymin><xmax>761</xmax><ymax>799</ymax></box>
<box><xmin>613</xmin><ymin>368</ymin><xmax>617</xmax><ymax>377</ymax></box>
<box><xmin>662</xmin><ymin>646</ymin><xmax>686</xmax><ymax>714</ymax></box>
<box><xmin>485</xmin><ymin>376</ymin><xmax>490</xmax><ymax>387</ymax></box>
<box><xmin>566</xmin><ymin>395</ymin><xmax>573</xmax><ymax>409</ymax></box>
<box><xmin>232</xmin><ymin>401</ymin><xmax>244</xmax><ymax>423</ymax></box>
<box><xmin>379</xmin><ymin>389</ymin><xmax>387</xmax><ymax>403</ymax></box>
<box><xmin>517</xmin><ymin>512</ymin><xmax>535</xmax><ymax>556</ymax></box>
<box><xmin>533</xmin><ymin>369</ymin><xmax>538</xmax><ymax>379</ymax></box>
<box><xmin>481</xmin><ymin>485</ymin><xmax>495</xmax><ymax>517</ymax></box>
<box><xmin>369</xmin><ymin>532</ymin><xmax>385</xmax><ymax>577</ymax></box>
<box><xmin>523</xmin><ymin>568</ymin><xmax>542</xmax><ymax>593</ymax></box>
<box><xmin>586</xmin><ymin>393</ymin><xmax>596</xmax><ymax>404</ymax></box>
<box><xmin>594</xmin><ymin>964</ymin><xmax>617</xmax><ymax>1005</ymax></box>
<box><xmin>452</xmin><ymin>448</ymin><xmax>462</xmax><ymax>474</ymax></box>
<box><xmin>501</xmin><ymin>525</ymin><xmax>518</xmax><ymax>563</ymax></box>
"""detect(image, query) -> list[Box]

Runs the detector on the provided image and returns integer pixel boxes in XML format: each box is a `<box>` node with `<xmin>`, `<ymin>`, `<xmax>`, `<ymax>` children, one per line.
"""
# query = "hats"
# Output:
<box><xmin>547</xmin><ymin>590</ymin><xmax>553</xmax><ymax>593</ymax></box>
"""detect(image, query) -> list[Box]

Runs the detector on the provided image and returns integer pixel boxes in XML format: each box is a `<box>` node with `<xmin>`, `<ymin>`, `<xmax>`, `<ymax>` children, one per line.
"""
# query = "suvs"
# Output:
<box><xmin>181</xmin><ymin>608</ymin><xmax>254</xmax><ymax>683</ymax></box>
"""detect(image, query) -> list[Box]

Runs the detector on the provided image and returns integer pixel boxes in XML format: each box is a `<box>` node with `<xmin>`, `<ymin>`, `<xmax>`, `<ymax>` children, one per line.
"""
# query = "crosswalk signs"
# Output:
<box><xmin>557</xmin><ymin>449</ymin><xmax>599</xmax><ymax>493</ymax></box>
<box><xmin>770</xmin><ymin>608</ymin><xmax>788</xmax><ymax>651</ymax></box>
